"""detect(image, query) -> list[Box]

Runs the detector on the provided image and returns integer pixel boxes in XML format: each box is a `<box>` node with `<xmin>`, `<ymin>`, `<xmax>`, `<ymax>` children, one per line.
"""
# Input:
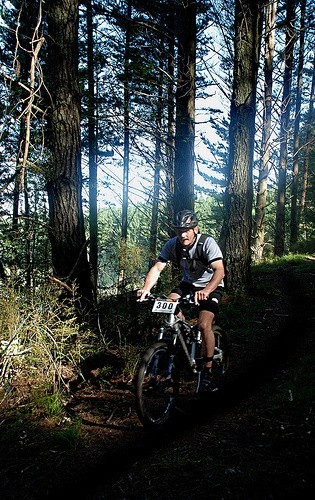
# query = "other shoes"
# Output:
<box><xmin>199</xmin><ymin>370</ymin><xmax>217</xmax><ymax>392</ymax></box>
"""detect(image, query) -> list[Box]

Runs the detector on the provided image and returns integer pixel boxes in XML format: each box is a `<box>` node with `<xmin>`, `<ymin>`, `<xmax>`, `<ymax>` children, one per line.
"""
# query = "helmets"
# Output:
<box><xmin>173</xmin><ymin>209</ymin><xmax>199</xmax><ymax>228</ymax></box>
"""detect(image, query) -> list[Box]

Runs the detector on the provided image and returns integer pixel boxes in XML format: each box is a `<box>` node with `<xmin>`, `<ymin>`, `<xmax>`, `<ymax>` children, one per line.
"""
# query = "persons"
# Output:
<box><xmin>136</xmin><ymin>210</ymin><xmax>225</xmax><ymax>388</ymax></box>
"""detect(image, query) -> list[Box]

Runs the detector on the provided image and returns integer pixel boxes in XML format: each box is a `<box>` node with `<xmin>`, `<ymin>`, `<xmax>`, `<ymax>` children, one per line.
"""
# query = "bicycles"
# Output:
<box><xmin>134</xmin><ymin>293</ymin><xmax>231</xmax><ymax>428</ymax></box>
